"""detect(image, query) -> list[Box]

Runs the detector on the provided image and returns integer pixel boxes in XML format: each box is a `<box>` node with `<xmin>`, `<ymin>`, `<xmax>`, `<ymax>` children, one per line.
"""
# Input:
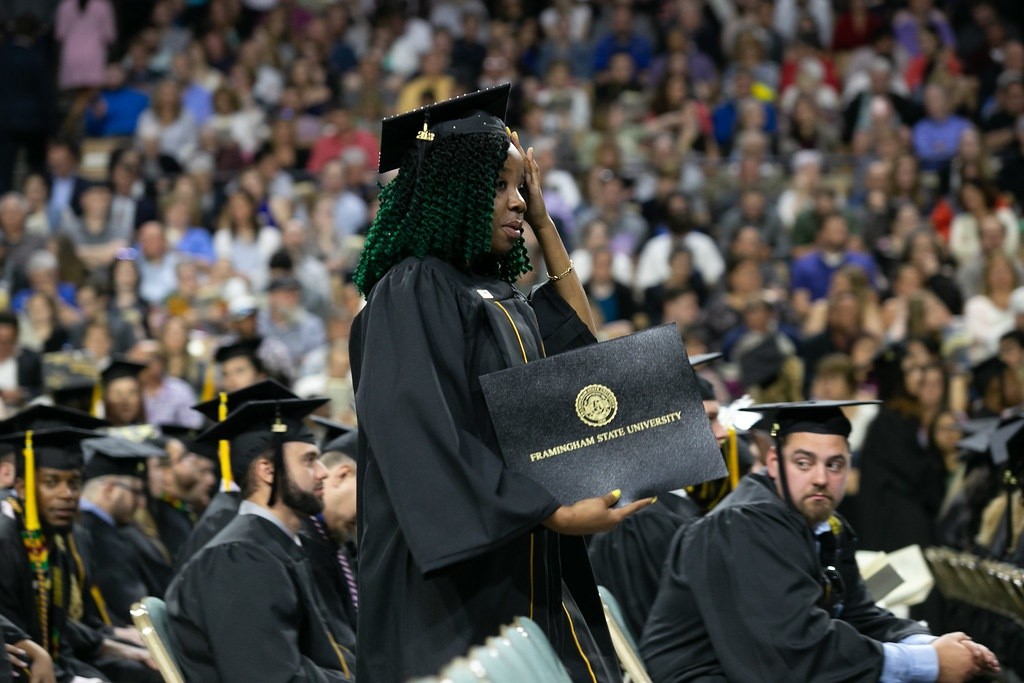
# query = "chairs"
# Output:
<box><xmin>129</xmin><ymin>597</ymin><xmax>185</xmax><ymax>682</ymax></box>
<box><xmin>418</xmin><ymin>586</ymin><xmax>655</xmax><ymax>682</ymax></box>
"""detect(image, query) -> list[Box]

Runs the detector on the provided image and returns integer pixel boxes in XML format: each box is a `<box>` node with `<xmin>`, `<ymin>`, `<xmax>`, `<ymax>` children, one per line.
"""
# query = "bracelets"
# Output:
<box><xmin>545</xmin><ymin>259</ymin><xmax>574</xmax><ymax>280</ymax></box>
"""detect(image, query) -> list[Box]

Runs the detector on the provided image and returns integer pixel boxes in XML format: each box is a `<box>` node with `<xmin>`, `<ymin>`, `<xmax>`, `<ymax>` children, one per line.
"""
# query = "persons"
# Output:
<box><xmin>588</xmin><ymin>352</ymin><xmax>730</xmax><ymax>642</ymax></box>
<box><xmin>712</xmin><ymin>70</ymin><xmax>776</xmax><ymax>142</ymax></box>
<box><xmin>0</xmin><ymin>0</ymin><xmax>1024</xmax><ymax>683</ymax></box>
<box><xmin>298</xmin><ymin>428</ymin><xmax>362</xmax><ymax>630</ymax></box>
<box><xmin>643</xmin><ymin>246</ymin><xmax>708</xmax><ymax>327</ymax></box>
<box><xmin>348</xmin><ymin>83</ymin><xmax>656</xmax><ymax>683</ymax></box>
<box><xmin>581</xmin><ymin>251</ymin><xmax>634</xmax><ymax>322</ymax></box>
<box><xmin>155</xmin><ymin>192</ymin><xmax>215</xmax><ymax>265</ymax></box>
<box><xmin>281</xmin><ymin>216</ymin><xmax>332</xmax><ymax>296</ymax></box>
<box><xmin>911</xmin><ymin>81</ymin><xmax>977</xmax><ymax>170</ymax></box>
<box><xmin>568</xmin><ymin>218</ymin><xmax>633</xmax><ymax>284</ymax></box>
<box><xmin>589</xmin><ymin>7</ymin><xmax>650</xmax><ymax>83</ymax></box>
<box><xmin>54</xmin><ymin>0</ymin><xmax>117</xmax><ymax>103</ymax></box>
<box><xmin>947</xmin><ymin>177</ymin><xmax>1020</xmax><ymax>264</ymax></box>
<box><xmin>164</xmin><ymin>397</ymin><xmax>357</xmax><ymax>683</ymax></box>
<box><xmin>35</xmin><ymin>144</ymin><xmax>89</xmax><ymax>232</ymax></box>
<box><xmin>638</xmin><ymin>400</ymin><xmax>1002</xmax><ymax>683</ymax></box>
<box><xmin>792</xmin><ymin>213</ymin><xmax>878</xmax><ymax>311</ymax></box>
<box><xmin>306</xmin><ymin>102</ymin><xmax>379</xmax><ymax>171</ymax></box>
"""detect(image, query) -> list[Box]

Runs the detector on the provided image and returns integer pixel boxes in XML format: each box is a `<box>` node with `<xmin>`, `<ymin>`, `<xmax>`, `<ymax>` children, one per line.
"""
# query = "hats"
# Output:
<box><xmin>100</xmin><ymin>360</ymin><xmax>147</xmax><ymax>383</ymax></box>
<box><xmin>185</xmin><ymin>379</ymin><xmax>360</xmax><ymax>509</ymax></box>
<box><xmin>214</xmin><ymin>336</ymin><xmax>262</xmax><ymax>361</ymax></box>
<box><xmin>737</xmin><ymin>397</ymin><xmax>883</xmax><ymax>438</ymax></box>
<box><xmin>688</xmin><ymin>351</ymin><xmax>723</xmax><ymax>417</ymax></box>
<box><xmin>0</xmin><ymin>404</ymin><xmax>113</xmax><ymax>534</ymax></box>
<box><xmin>377</xmin><ymin>82</ymin><xmax>514</xmax><ymax>177</ymax></box>
